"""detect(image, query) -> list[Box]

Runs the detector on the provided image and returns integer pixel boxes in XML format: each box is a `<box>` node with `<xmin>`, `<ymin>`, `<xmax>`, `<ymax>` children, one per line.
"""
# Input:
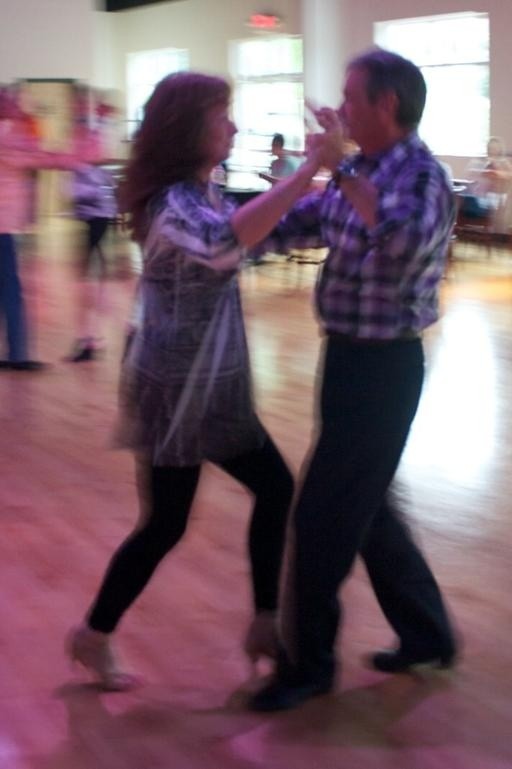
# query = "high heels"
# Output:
<box><xmin>243</xmin><ymin>632</ymin><xmax>282</xmax><ymax>664</ymax></box>
<box><xmin>63</xmin><ymin>628</ymin><xmax>139</xmax><ymax>691</ymax></box>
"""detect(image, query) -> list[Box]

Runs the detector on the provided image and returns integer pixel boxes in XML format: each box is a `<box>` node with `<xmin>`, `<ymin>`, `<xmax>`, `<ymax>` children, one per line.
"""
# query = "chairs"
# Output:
<box><xmin>458</xmin><ymin>193</ymin><xmax>493</xmax><ymax>258</ymax></box>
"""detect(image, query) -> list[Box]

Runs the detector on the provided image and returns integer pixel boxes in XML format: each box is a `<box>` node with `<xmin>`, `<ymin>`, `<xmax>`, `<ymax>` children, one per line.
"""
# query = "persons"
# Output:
<box><xmin>248</xmin><ymin>134</ymin><xmax>298</xmax><ymax>262</ymax></box>
<box><xmin>242</xmin><ymin>49</ymin><xmax>457</xmax><ymax>711</ymax></box>
<box><xmin>62</xmin><ymin>71</ymin><xmax>350</xmax><ymax>691</ymax></box>
<box><xmin>447</xmin><ymin>136</ymin><xmax>512</xmax><ymax>260</ymax></box>
<box><xmin>0</xmin><ymin>88</ymin><xmax>145</xmax><ymax>370</ymax></box>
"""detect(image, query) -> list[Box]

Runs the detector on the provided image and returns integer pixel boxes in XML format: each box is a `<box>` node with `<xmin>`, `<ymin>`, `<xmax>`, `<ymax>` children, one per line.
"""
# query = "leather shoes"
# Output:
<box><xmin>244</xmin><ymin>677</ymin><xmax>332</xmax><ymax>714</ymax></box>
<box><xmin>67</xmin><ymin>340</ymin><xmax>100</xmax><ymax>360</ymax></box>
<box><xmin>371</xmin><ymin>642</ymin><xmax>457</xmax><ymax>669</ymax></box>
<box><xmin>0</xmin><ymin>357</ymin><xmax>45</xmax><ymax>371</ymax></box>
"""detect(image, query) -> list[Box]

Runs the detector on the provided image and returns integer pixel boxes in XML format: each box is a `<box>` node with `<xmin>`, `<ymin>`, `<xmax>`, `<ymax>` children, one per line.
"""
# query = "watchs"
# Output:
<box><xmin>332</xmin><ymin>158</ymin><xmax>358</xmax><ymax>188</ymax></box>
